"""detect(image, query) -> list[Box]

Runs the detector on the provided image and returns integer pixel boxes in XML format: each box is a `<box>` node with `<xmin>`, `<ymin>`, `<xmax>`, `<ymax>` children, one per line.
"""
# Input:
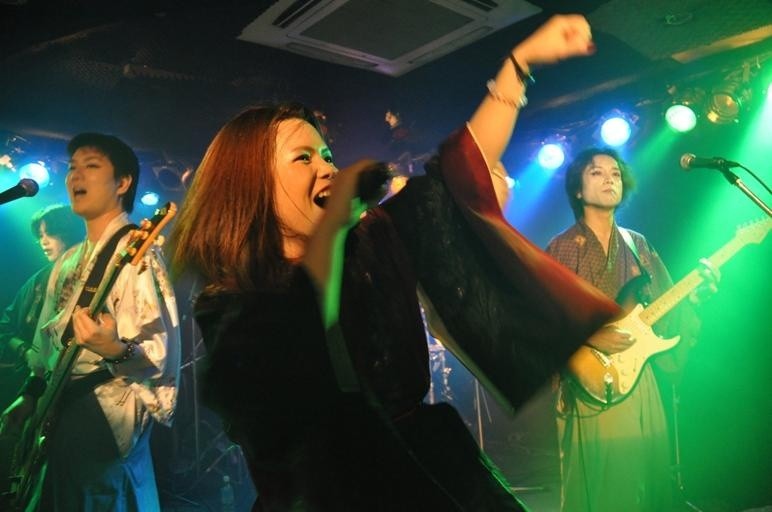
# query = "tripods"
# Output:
<box><xmin>159</xmin><ymin>312</ymin><xmax>250</xmax><ymax>507</ymax></box>
<box><xmin>421</xmin><ymin>306</ymin><xmax>551</xmax><ymax>495</ymax></box>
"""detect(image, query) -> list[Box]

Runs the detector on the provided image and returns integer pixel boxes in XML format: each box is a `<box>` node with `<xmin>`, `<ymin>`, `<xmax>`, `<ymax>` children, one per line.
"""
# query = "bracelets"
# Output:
<box><xmin>15</xmin><ymin>341</ymin><xmax>31</xmax><ymax>362</ymax></box>
<box><xmin>16</xmin><ymin>375</ymin><xmax>46</xmax><ymax>399</ymax></box>
<box><xmin>484</xmin><ymin>76</ymin><xmax>530</xmax><ymax>110</ymax></box>
<box><xmin>101</xmin><ymin>338</ymin><xmax>138</xmax><ymax>363</ymax></box>
<box><xmin>503</xmin><ymin>50</ymin><xmax>537</xmax><ymax>86</ymax></box>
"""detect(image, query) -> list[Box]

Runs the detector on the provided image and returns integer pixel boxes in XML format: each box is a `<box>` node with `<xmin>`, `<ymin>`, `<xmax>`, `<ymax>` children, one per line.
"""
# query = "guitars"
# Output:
<box><xmin>9</xmin><ymin>201</ymin><xmax>177</xmax><ymax>512</ymax></box>
<box><xmin>559</xmin><ymin>213</ymin><xmax>770</xmax><ymax>408</ymax></box>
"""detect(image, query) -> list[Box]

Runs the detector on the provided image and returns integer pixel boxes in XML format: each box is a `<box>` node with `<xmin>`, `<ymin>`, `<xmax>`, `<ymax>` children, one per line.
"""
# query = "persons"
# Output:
<box><xmin>166</xmin><ymin>10</ymin><xmax>595</xmax><ymax>510</ymax></box>
<box><xmin>0</xmin><ymin>203</ymin><xmax>88</xmax><ymax>362</ymax></box>
<box><xmin>543</xmin><ymin>146</ymin><xmax>726</xmax><ymax>510</ymax></box>
<box><xmin>0</xmin><ymin>133</ymin><xmax>185</xmax><ymax>510</ymax></box>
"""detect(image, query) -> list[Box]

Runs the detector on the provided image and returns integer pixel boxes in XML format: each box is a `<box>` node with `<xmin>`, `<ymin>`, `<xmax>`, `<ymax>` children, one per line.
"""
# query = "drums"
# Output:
<box><xmin>422</xmin><ymin>343</ymin><xmax>450</xmax><ymax>404</ymax></box>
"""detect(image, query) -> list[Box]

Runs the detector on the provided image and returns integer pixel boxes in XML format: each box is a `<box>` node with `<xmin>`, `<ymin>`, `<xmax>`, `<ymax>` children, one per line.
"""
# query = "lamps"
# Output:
<box><xmin>535</xmin><ymin>58</ymin><xmax>760</xmax><ymax>174</ymax></box>
<box><xmin>0</xmin><ymin>148</ymin><xmax>197</xmax><ymax>208</ymax></box>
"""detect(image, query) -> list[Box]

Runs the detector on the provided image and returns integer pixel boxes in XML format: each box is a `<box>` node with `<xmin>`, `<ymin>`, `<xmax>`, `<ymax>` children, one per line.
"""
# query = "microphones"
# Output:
<box><xmin>0</xmin><ymin>179</ymin><xmax>39</xmax><ymax>204</ymax></box>
<box><xmin>680</xmin><ymin>153</ymin><xmax>737</xmax><ymax>170</ymax></box>
<box><xmin>358</xmin><ymin>162</ymin><xmax>390</xmax><ymax>201</ymax></box>
